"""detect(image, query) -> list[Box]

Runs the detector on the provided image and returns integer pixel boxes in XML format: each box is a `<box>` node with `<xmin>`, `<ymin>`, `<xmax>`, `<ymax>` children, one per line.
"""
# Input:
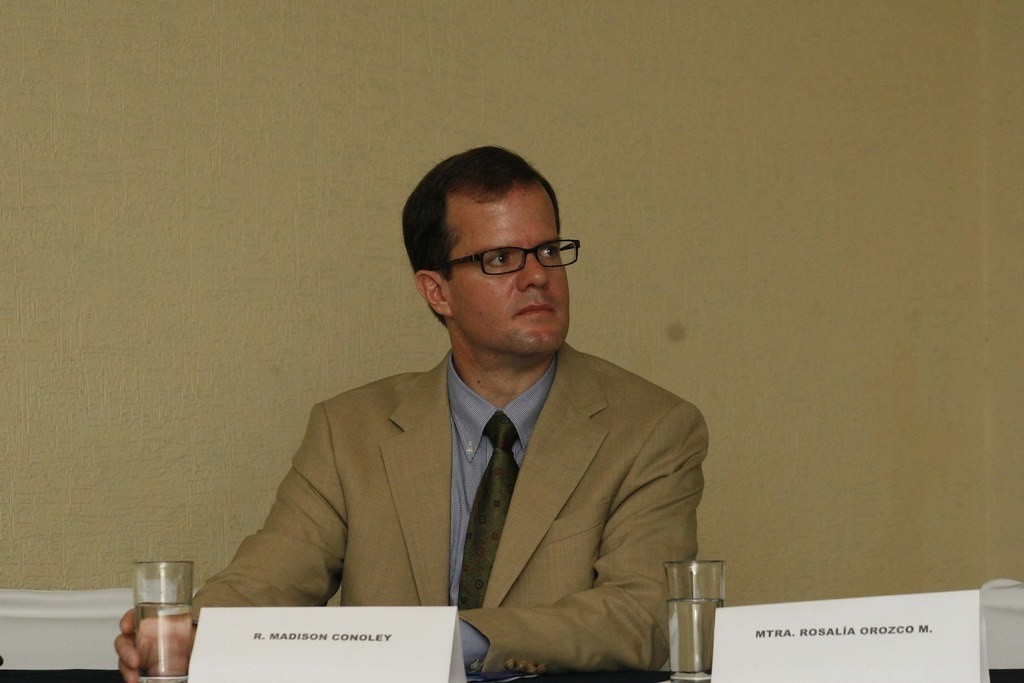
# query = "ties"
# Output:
<box><xmin>457</xmin><ymin>410</ymin><xmax>521</xmax><ymax>612</ymax></box>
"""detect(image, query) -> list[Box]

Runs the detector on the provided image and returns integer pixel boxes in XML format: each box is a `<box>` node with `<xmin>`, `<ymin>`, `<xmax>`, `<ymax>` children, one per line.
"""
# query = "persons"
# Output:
<box><xmin>114</xmin><ymin>147</ymin><xmax>709</xmax><ymax>683</ymax></box>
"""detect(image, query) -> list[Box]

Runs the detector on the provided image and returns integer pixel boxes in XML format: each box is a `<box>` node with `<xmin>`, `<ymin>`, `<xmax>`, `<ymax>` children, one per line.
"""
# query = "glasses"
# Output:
<box><xmin>431</xmin><ymin>239</ymin><xmax>580</xmax><ymax>275</ymax></box>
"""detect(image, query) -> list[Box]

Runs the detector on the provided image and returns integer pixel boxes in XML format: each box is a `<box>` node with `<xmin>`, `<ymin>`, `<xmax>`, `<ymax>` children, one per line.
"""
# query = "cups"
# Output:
<box><xmin>664</xmin><ymin>560</ymin><xmax>725</xmax><ymax>683</ymax></box>
<box><xmin>131</xmin><ymin>561</ymin><xmax>193</xmax><ymax>683</ymax></box>
